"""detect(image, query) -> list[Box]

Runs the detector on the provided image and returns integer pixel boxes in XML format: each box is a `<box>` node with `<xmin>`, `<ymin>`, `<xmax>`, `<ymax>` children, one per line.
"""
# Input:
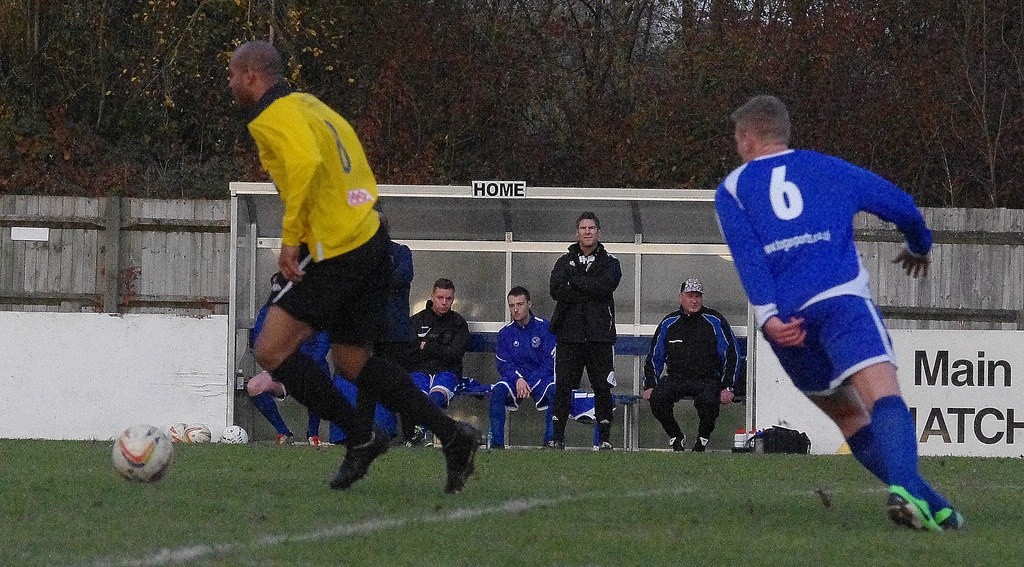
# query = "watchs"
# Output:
<box><xmin>726</xmin><ymin>387</ymin><xmax>734</xmax><ymax>392</ymax></box>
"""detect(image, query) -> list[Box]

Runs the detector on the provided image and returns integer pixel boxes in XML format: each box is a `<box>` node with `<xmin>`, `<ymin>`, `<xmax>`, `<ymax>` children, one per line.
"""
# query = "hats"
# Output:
<box><xmin>680</xmin><ymin>277</ymin><xmax>704</xmax><ymax>293</ymax></box>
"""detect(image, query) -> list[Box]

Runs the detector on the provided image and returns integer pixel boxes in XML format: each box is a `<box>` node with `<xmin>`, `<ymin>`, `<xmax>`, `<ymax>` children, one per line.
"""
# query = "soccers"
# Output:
<box><xmin>166</xmin><ymin>423</ymin><xmax>190</xmax><ymax>443</ymax></box>
<box><xmin>219</xmin><ymin>426</ymin><xmax>249</xmax><ymax>444</ymax></box>
<box><xmin>181</xmin><ymin>423</ymin><xmax>212</xmax><ymax>445</ymax></box>
<box><xmin>111</xmin><ymin>424</ymin><xmax>176</xmax><ymax>483</ymax></box>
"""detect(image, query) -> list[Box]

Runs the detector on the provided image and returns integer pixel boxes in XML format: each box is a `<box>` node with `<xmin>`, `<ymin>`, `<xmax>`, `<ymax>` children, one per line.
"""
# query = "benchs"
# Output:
<box><xmin>457</xmin><ymin>336</ymin><xmax>745</xmax><ymax>452</ymax></box>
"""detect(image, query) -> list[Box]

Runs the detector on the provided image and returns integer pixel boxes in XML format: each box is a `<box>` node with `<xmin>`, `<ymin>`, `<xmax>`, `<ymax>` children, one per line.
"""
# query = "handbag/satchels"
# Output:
<box><xmin>746</xmin><ymin>425</ymin><xmax>811</xmax><ymax>456</ymax></box>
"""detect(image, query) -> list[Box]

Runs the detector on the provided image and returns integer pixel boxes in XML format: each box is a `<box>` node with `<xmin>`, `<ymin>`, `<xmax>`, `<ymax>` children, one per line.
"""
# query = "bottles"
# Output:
<box><xmin>734</xmin><ymin>429</ymin><xmax>747</xmax><ymax>448</ymax></box>
<box><xmin>749</xmin><ymin>427</ymin><xmax>755</xmax><ymax>447</ymax></box>
<box><xmin>755</xmin><ymin>431</ymin><xmax>764</xmax><ymax>454</ymax></box>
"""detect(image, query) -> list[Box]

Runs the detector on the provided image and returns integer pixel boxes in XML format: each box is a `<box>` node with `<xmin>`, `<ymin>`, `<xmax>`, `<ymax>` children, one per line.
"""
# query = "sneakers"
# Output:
<box><xmin>668</xmin><ymin>432</ymin><xmax>687</xmax><ymax>453</ymax></box>
<box><xmin>592</xmin><ymin>439</ymin><xmax>614</xmax><ymax>452</ymax></box>
<box><xmin>308</xmin><ymin>436</ymin><xmax>323</xmax><ymax>448</ymax></box>
<box><xmin>885</xmin><ymin>486</ymin><xmax>941</xmax><ymax>533</ymax></box>
<box><xmin>272</xmin><ymin>432</ymin><xmax>294</xmax><ymax>447</ymax></box>
<box><xmin>331</xmin><ymin>433</ymin><xmax>388</xmax><ymax>491</ymax></box>
<box><xmin>405</xmin><ymin>426</ymin><xmax>424</xmax><ymax>447</ymax></box>
<box><xmin>540</xmin><ymin>440</ymin><xmax>565</xmax><ymax>451</ymax></box>
<box><xmin>690</xmin><ymin>435</ymin><xmax>708</xmax><ymax>452</ymax></box>
<box><xmin>931</xmin><ymin>506</ymin><xmax>962</xmax><ymax>531</ymax></box>
<box><xmin>444</xmin><ymin>421</ymin><xmax>482</xmax><ymax>498</ymax></box>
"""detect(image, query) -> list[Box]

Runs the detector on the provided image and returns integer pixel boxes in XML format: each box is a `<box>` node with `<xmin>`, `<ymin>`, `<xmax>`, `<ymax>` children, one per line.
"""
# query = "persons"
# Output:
<box><xmin>230</xmin><ymin>39</ymin><xmax>487</xmax><ymax>496</ymax></box>
<box><xmin>486</xmin><ymin>285</ymin><xmax>557</xmax><ymax>452</ymax></box>
<box><xmin>244</xmin><ymin>271</ymin><xmax>400</xmax><ymax>447</ymax></box>
<box><xmin>408</xmin><ymin>277</ymin><xmax>471</xmax><ymax>450</ymax></box>
<box><xmin>641</xmin><ymin>277</ymin><xmax>741</xmax><ymax>452</ymax></box>
<box><xmin>712</xmin><ymin>95</ymin><xmax>967</xmax><ymax>536</ymax></box>
<box><xmin>544</xmin><ymin>211</ymin><xmax>622</xmax><ymax>452</ymax></box>
